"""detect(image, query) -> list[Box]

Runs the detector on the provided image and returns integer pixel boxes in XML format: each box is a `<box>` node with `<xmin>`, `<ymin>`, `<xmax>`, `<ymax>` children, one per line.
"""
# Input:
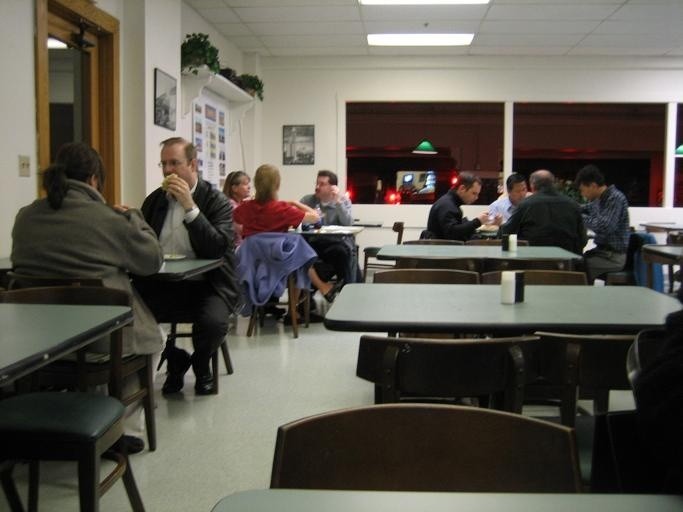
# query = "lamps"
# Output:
<box><xmin>368</xmin><ymin>32</ymin><xmax>474</xmax><ymax>48</ymax></box>
<box><xmin>411</xmin><ymin>140</ymin><xmax>437</xmax><ymax>156</ymax></box>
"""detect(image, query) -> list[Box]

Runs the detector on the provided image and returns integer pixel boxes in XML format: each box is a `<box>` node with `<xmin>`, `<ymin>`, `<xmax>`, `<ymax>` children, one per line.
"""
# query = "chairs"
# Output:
<box><xmin>270</xmin><ymin>402</ymin><xmax>582</xmax><ymax>493</ymax></box>
<box><xmin>482</xmin><ymin>270</ymin><xmax>588</xmax><ymax>287</ymax></box>
<box><xmin>535</xmin><ymin>331</ymin><xmax>637</xmax><ymax>426</ymax></box>
<box><xmin>372</xmin><ymin>269</ymin><xmax>479</xmax><ymax>285</ymax></box>
<box><xmin>362</xmin><ymin>221</ymin><xmax>404</xmax><ymax>282</ymax></box>
<box><xmin>355</xmin><ymin>335</ymin><xmax>541</xmax><ymax>414</ymax></box>
<box><xmin>1</xmin><ymin>271</ymin><xmax>157</xmax><ymax>453</ymax></box>
<box><xmin>246</xmin><ymin>234</ymin><xmax>311</xmax><ymax>339</ymax></box>
<box><xmin>0</xmin><ymin>286</ymin><xmax>145</xmax><ymax>511</ymax></box>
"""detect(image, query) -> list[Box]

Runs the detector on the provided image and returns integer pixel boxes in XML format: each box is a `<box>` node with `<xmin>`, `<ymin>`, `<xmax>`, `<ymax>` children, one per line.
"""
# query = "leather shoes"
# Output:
<box><xmin>324</xmin><ymin>279</ymin><xmax>344</xmax><ymax>303</ymax></box>
<box><xmin>114</xmin><ymin>435</ymin><xmax>144</xmax><ymax>451</ymax></box>
<box><xmin>191</xmin><ymin>353</ymin><xmax>213</xmax><ymax>394</ymax></box>
<box><xmin>283</xmin><ymin>313</ymin><xmax>323</xmax><ymax>326</ymax></box>
<box><xmin>161</xmin><ymin>349</ymin><xmax>190</xmax><ymax>394</ymax></box>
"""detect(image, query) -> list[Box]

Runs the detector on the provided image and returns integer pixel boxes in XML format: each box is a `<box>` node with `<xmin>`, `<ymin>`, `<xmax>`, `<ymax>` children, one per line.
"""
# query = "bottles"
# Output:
<box><xmin>502</xmin><ymin>234</ymin><xmax>509</xmax><ymax>252</ymax></box>
<box><xmin>509</xmin><ymin>234</ymin><xmax>517</xmax><ymax>251</ymax></box>
<box><xmin>501</xmin><ymin>270</ymin><xmax>516</xmax><ymax>304</ymax></box>
<box><xmin>314</xmin><ymin>204</ymin><xmax>321</xmax><ymax>229</ymax></box>
<box><xmin>515</xmin><ymin>271</ymin><xmax>525</xmax><ymax>302</ymax></box>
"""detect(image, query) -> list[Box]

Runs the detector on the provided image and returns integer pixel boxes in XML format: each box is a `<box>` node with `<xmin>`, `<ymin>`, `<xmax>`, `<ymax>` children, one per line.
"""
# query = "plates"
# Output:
<box><xmin>164</xmin><ymin>254</ymin><xmax>185</xmax><ymax>259</ymax></box>
<box><xmin>479</xmin><ymin>228</ymin><xmax>498</xmax><ymax>232</ymax></box>
<box><xmin>288</xmin><ymin>229</ymin><xmax>295</xmax><ymax>231</ymax></box>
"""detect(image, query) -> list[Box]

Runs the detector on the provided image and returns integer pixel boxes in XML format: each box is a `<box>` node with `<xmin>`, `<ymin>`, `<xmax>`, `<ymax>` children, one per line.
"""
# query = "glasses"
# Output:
<box><xmin>157</xmin><ymin>158</ymin><xmax>187</xmax><ymax>167</ymax></box>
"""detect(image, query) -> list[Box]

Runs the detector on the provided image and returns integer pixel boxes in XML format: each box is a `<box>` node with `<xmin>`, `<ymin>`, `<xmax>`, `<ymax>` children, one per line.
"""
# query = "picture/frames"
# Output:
<box><xmin>153</xmin><ymin>67</ymin><xmax>177</xmax><ymax>130</ymax></box>
<box><xmin>281</xmin><ymin>124</ymin><xmax>315</xmax><ymax>165</ymax></box>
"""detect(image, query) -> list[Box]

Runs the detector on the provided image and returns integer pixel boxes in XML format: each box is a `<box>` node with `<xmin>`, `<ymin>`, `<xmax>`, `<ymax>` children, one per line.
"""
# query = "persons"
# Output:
<box><xmin>498</xmin><ymin>168</ymin><xmax>587</xmax><ymax>261</ymax></box>
<box><xmin>222</xmin><ymin>170</ymin><xmax>252</xmax><ymax>244</ymax></box>
<box><xmin>140</xmin><ymin>137</ymin><xmax>238</xmax><ymax>397</ymax></box>
<box><xmin>232</xmin><ymin>163</ymin><xmax>344</xmax><ymax>327</ymax></box>
<box><xmin>571</xmin><ymin>166</ymin><xmax>630</xmax><ymax>285</ymax></box>
<box><xmin>427</xmin><ymin>171</ymin><xmax>494</xmax><ymax>241</ymax></box>
<box><xmin>297</xmin><ymin>169</ymin><xmax>358</xmax><ymax>286</ymax></box>
<box><xmin>10</xmin><ymin>139</ymin><xmax>164</xmax><ymax>455</ymax></box>
<box><xmin>485</xmin><ymin>173</ymin><xmax>530</xmax><ymax>231</ymax></box>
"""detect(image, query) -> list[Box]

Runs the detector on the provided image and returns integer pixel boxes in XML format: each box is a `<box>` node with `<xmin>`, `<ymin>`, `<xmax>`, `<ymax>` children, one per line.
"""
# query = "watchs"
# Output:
<box><xmin>183</xmin><ymin>204</ymin><xmax>198</xmax><ymax>214</ymax></box>
